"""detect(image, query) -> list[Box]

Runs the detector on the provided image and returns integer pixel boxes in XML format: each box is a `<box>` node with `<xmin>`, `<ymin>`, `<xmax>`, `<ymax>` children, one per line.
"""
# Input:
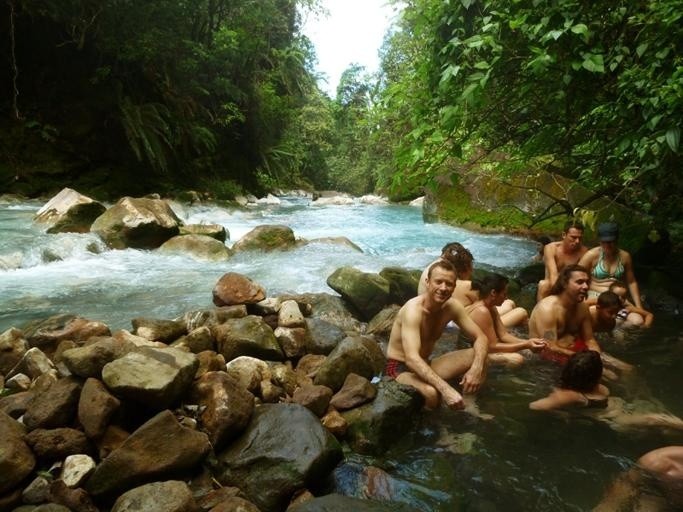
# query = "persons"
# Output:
<box><xmin>578</xmin><ymin>221</ymin><xmax>643</xmax><ymax>331</ymax></box>
<box><xmin>418</xmin><ymin>242</ymin><xmax>464</xmax><ymax>296</ymax></box>
<box><xmin>584</xmin><ymin>280</ymin><xmax>654</xmax><ymax>331</ymax></box>
<box><xmin>528</xmin><ymin>350</ymin><xmax>610</xmax><ymax>411</ymax></box>
<box><xmin>529</xmin><ymin>234</ymin><xmax>552</xmax><ymax>264</ymax></box>
<box><xmin>528</xmin><ymin>265</ymin><xmax>635</xmax><ymax>383</ymax></box>
<box><xmin>536</xmin><ymin>221</ymin><xmax>589</xmax><ymax>302</ymax></box>
<box><xmin>591</xmin><ymin>446</ymin><xmax>683</xmax><ymax>512</ymax></box>
<box><xmin>384</xmin><ymin>260</ymin><xmax>488</xmax><ymax>412</ymax></box>
<box><xmin>446</xmin><ymin>251</ymin><xmax>528</xmax><ymax>328</ymax></box>
<box><xmin>590</xmin><ymin>292</ymin><xmax>624</xmax><ymax>339</ymax></box>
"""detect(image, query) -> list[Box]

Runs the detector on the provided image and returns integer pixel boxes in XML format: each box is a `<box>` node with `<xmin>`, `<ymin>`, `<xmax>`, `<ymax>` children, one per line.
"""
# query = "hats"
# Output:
<box><xmin>596</xmin><ymin>223</ymin><xmax>619</xmax><ymax>243</ymax></box>
